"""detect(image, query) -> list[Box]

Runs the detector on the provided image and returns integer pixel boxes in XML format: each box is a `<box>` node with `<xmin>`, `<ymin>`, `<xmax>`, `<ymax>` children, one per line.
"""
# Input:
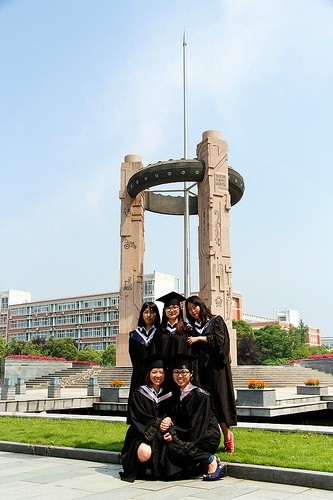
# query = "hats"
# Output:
<box><xmin>171</xmin><ymin>353</ymin><xmax>198</xmax><ymax>369</ymax></box>
<box><xmin>154</xmin><ymin>291</ymin><xmax>186</xmax><ymax>306</ymax></box>
<box><xmin>149</xmin><ymin>354</ymin><xmax>166</xmax><ymax>368</ymax></box>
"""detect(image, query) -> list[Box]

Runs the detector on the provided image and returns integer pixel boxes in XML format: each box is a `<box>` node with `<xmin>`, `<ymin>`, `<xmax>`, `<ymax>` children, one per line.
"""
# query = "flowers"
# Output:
<box><xmin>290</xmin><ymin>359</ymin><xmax>300</xmax><ymax>363</ymax></box>
<box><xmin>304</xmin><ymin>378</ymin><xmax>320</xmax><ymax>386</ymax></box>
<box><xmin>112</xmin><ymin>379</ymin><xmax>125</xmax><ymax>387</ymax></box>
<box><xmin>308</xmin><ymin>354</ymin><xmax>333</xmax><ymax>359</ymax></box>
<box><xmin>246</xmin><ymin>379</ymin><xmax>265</xmax><ymax>389</ymax></box>
<box><xmin>6</xmin><ymin>354</ymin><xmax>97</xmax><ymax>365</ymax></box>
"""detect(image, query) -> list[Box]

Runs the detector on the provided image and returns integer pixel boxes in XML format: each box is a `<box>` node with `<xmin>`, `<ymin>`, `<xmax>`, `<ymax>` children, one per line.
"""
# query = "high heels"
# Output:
<box><xmin>203</xmin><ymin>455</ymin><xmax>227</xmax><ymax>481</ymax></box>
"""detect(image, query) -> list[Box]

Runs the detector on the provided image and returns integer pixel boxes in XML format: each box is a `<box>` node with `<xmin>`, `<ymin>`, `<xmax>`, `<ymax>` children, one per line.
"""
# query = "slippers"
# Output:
<box><xmin>223</xmin><ymin>432</ymin><xmax>234</xmax><ymax>453</ymax></box>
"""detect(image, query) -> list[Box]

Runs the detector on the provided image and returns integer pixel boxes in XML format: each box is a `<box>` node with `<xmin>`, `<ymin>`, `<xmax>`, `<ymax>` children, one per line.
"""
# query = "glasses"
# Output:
<box><xmin>172</xmin><ymin>371</ymin><xmax>189</xmax><ymax>376</ymax></box>
<box><xmin>164</xmin><ymin>307</ymin><xmax>179</xmax><ymax>311</ymax></box>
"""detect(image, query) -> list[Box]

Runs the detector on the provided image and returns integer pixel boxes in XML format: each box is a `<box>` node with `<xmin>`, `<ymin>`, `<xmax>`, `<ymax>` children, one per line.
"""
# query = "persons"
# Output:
<box><xmin>164</xmin><ymin>361</ymin><xmax>227</xmax><ymax>481</ymax></box>
<box><xmin>119</xmin><ymin>359</ymin><xmax>170</xmax><ymax>483</ymax></box>
<box><xmin>185</xmin><ymin>296</ymin><xmax>237</xmax><ymax>452</ymax></box>
<box><xmin>155</xmin><ymin>291</ymin><xmax>194</xmax><ymax>387</ymax></box>
<box><xmin>126</xmin><ymin>302</ymin><xmax>161</xmax><ymax>426</ymax></box>
